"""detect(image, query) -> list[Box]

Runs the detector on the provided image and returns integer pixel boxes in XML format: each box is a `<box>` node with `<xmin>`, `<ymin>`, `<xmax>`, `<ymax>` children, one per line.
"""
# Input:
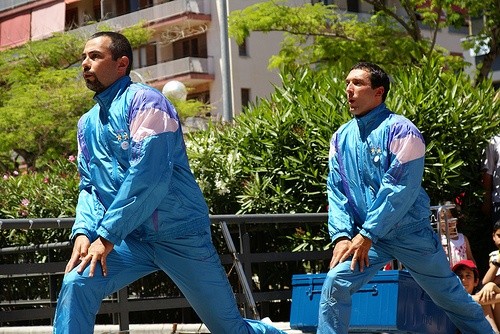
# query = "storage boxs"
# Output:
<box><xmin>290</xmin><ymin>270</ymin><xmax>447</xmax><ymax>334</ymax></box>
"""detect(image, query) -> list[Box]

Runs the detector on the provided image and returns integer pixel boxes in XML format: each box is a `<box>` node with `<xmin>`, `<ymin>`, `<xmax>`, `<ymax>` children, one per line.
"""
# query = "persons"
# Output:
<box><xmin>317</xmin><ymin>62</ymin><xmax>496</xmax><ymax>334</ymax></box>
<box><xmin>383</xmin><ymin>124</ymin><xmax>500</xmax><ymax>334</ymax></box>
<box><xmin>53</xmin><ymin>31</ymin><xmax>290</xmax><ymax>334</ymax></box>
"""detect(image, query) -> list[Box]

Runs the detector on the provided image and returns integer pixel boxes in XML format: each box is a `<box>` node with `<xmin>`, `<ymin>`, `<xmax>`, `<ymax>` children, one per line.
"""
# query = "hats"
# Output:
<box><xmin>451</xmin><ymin>260</ymin><xmax>479</xmax><ymax>276</ymax></box>
